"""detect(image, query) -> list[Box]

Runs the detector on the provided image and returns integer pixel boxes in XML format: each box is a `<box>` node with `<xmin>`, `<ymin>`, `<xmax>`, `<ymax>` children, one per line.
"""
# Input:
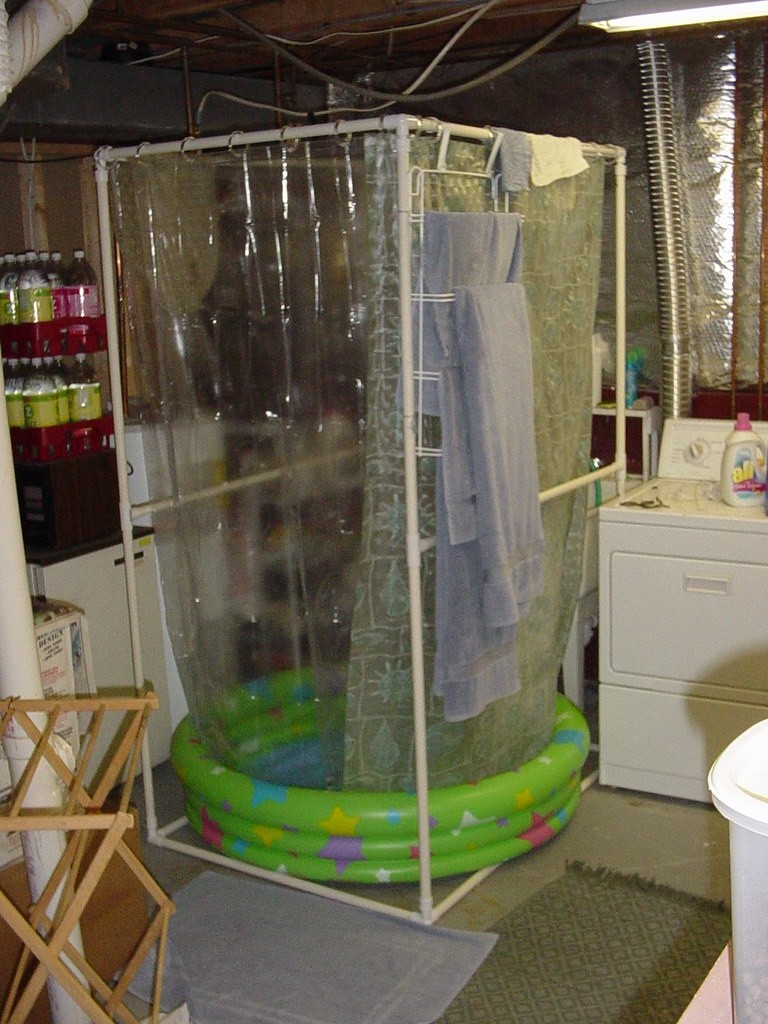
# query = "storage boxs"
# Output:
<box><xmin>0</xmin><ymin>798</ymin><xmax>147</xmax><ymax>1024</ymax></box>
<box><xmin>11</xmin><ymin>411</ymin><xmax>120</xmax><ymax>551</ymax></box>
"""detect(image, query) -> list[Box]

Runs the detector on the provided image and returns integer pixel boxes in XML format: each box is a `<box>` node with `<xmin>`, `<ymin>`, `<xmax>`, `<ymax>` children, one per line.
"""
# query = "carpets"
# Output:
<box><xmin>437</xmin><ymin>855</ymin><xmax>732</xmax><ymax>1024</ymax></box>
<box><xmin>111</xmin><ymin>868</ymin><xmax>498</xmax><ymax>1024</ymax></box>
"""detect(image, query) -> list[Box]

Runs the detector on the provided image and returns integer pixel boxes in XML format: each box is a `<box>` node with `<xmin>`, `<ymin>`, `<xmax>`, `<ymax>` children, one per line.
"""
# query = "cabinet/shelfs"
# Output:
<box><xmin>587</xmin><ymin>404</ymin><xmax>662</xmax><ymax>511</ymax></box>
<box><xmin>30</xmin><ymin>534</ymin><xmax>172</xmax><ymax>792</ymax></box>
<box><xmin>596</xmin><ymin>521</ymin><xmax>768</xmax><ymax>802</ymax></box>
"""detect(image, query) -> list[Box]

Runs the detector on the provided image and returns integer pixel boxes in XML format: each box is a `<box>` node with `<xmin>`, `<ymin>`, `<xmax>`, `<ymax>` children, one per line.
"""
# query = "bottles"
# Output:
<box><xmin>0</xmin><ymin>249</ymin><xmax>110</xmax><ymax>464</ymax></box>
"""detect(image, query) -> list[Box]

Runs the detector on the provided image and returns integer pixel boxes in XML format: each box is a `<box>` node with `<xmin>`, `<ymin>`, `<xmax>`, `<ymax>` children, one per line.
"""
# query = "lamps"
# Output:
<box><xmin>577</xmin><ymin>0</ymin><xmax>768</xmax><ymax>34</ymax></box>
